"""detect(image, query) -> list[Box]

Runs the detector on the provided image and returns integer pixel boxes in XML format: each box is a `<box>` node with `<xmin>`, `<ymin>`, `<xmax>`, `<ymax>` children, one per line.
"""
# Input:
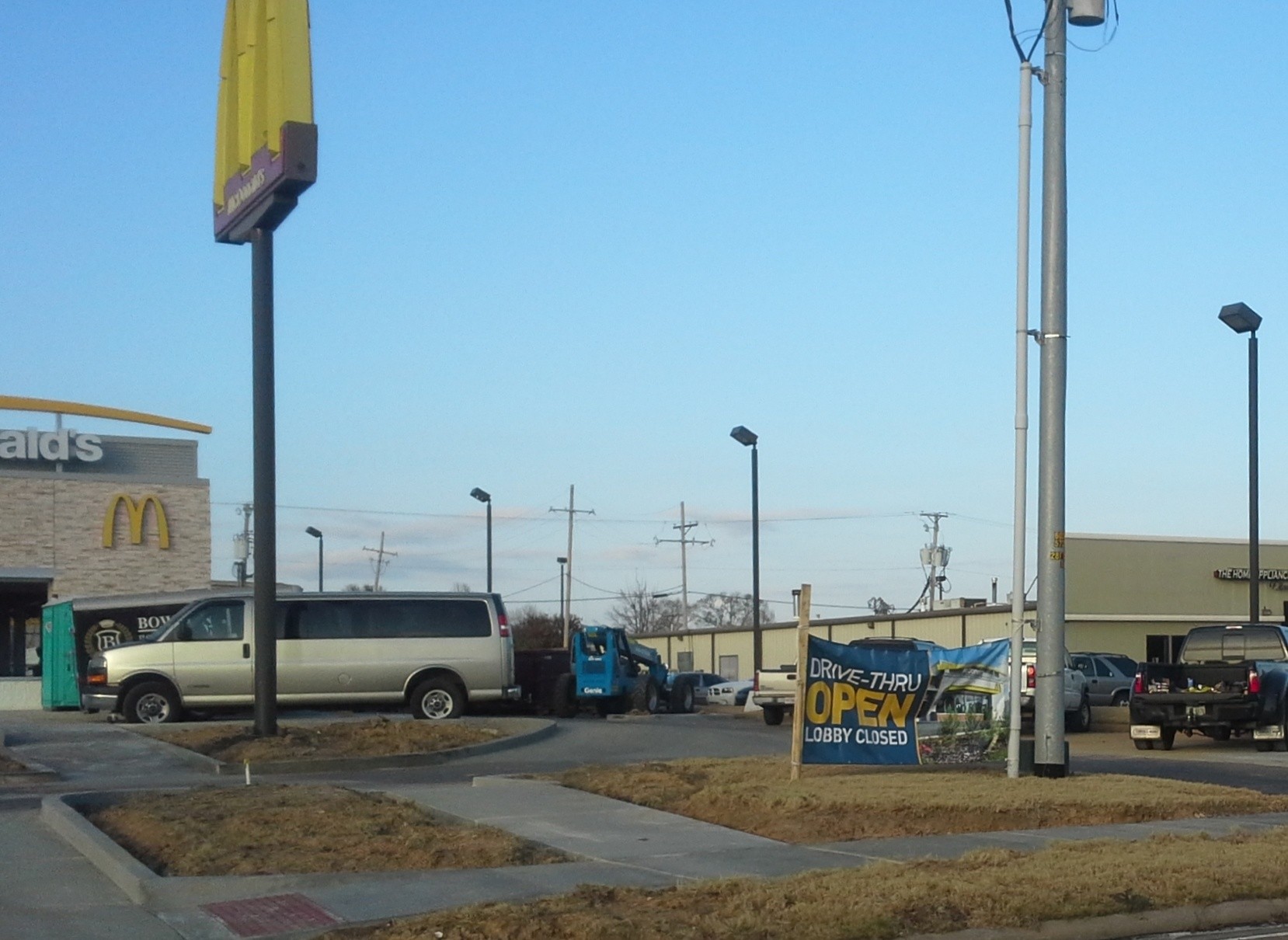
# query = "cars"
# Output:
<box><xmin>669</xmin><ymin>669</ymin><xmax>731</xmax><ymax>699</ymax></box>
<box><xmin>705</xmin><ymin>677</ymin><xmax>755</xmax><ymax>707</ymax></box>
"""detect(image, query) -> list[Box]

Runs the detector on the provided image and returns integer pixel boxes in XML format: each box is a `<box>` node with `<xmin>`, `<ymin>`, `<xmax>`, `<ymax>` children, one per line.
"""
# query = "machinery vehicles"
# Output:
<box><xmin>552</xmin><ymin>626</ymin><xmax>695</xmax><ymax>719</ymax></box>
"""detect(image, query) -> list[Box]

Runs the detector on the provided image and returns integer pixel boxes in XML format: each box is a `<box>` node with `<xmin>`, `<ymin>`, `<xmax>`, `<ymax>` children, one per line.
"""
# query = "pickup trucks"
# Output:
<box><xmin>1128</xmin><ymin>623</ymin><xmax>1288</xmax><ymax>751</ymax></box>
<box><xmin>753</xmin><ymin>657</ymin><xmax>798</xmax><ymax>726</ymax></box>
<box><xmin>977</xmin><ymin>637</ymin><xmax>1092</xmax><ymax>733</ymax></box>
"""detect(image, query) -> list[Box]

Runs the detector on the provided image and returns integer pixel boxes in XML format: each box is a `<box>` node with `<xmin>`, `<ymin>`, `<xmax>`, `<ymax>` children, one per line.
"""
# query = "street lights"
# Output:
<box><xmin>469</xmin><ymin>486</ymin><xmax>492</xmax><ymax>593</ymax></box>
<box><xmin>729</xmin><ymin>425</ymin><xmax>762</xmax><ymax>672</ymax></box>
<box><xmin>556</xmin><ymin>556</ymin><xmax>567</xmax><ymax>648</ymax></box>
<box><xmin>1217</xmin><ymin>300</ymin><xmax>1264</xmax><ymax>622</ymax></box>
<box><xmin>305</xmin><ymin>526</ymin><xmax>324</xmax><ymax>592</ymax></box>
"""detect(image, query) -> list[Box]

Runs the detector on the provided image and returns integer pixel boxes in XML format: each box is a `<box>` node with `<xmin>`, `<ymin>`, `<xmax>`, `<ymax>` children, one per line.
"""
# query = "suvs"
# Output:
<box><xmin>1070</xmin><ymin>652</ymin><xmax>1139</xmax><ymax>707</ymax></box>
<box><xmin>848</xmin><ymin>636</ymin><xmax>948</xmax><ymax>651</ymax></box>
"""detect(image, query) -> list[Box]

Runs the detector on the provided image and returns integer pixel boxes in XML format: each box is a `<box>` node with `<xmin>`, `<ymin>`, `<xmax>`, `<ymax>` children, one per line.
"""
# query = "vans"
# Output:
<box><xmin>80</xmin><ymin>591</ymin><xmax>521</xmax><ymax>724</ymax></box>
